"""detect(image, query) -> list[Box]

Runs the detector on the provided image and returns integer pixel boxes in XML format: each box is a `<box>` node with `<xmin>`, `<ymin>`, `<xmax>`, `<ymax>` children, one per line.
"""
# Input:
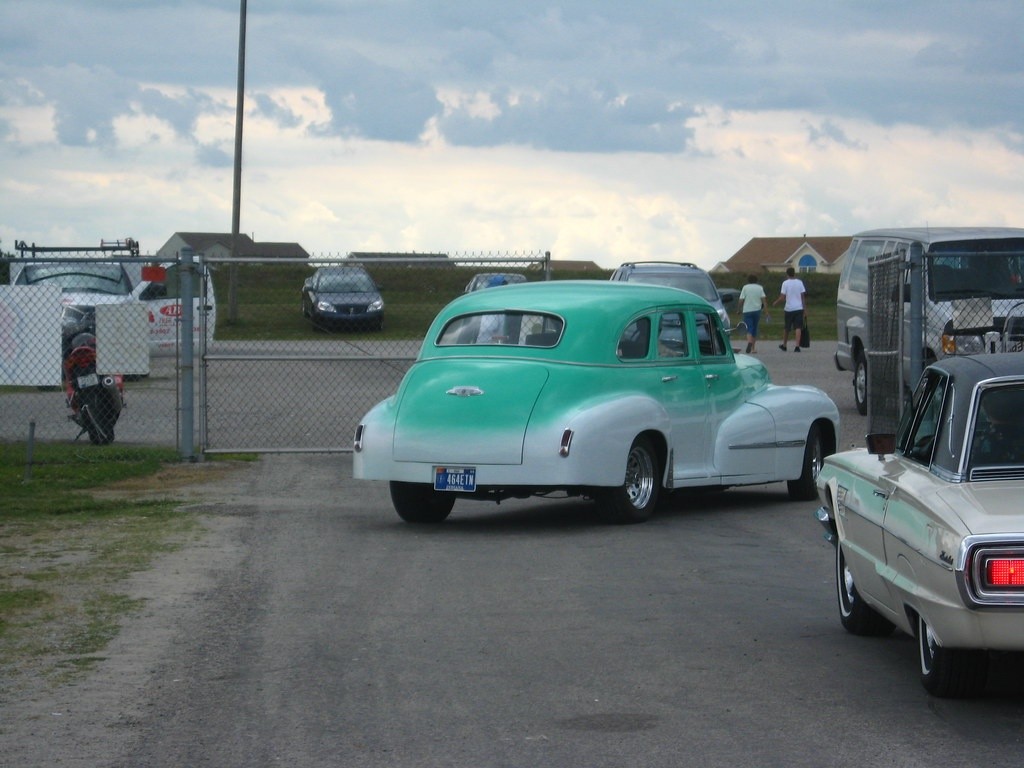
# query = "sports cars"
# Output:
<box><xmin>816</xmin><ymin>352</ymin><xmax>1024</xmax><ymax>698</ymax></box>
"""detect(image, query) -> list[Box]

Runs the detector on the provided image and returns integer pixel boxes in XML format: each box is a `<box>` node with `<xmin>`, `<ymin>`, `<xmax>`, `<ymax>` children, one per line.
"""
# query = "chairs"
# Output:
<box><xmin>933</xmin><ymin>264</ymin><xmax>977</xmax><ymax>295</ymax></box>
<box><xmin>526</xmin><ymin>333</ymin><xmax>644</xmax><ymax>360</ymax></box>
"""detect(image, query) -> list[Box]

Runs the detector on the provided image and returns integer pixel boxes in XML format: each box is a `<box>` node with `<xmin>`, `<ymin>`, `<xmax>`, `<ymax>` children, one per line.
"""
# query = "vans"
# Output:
<box><xmin>834</xmin><ymin>224</ymin><xmax>1024</xmax><ymax>415</ymax></box>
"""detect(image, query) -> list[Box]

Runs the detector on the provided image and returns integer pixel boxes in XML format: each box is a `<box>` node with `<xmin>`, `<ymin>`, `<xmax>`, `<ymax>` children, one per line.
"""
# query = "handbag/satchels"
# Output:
<box><xmin>799</xmin><ymin>316</ymin><xmax>810</xmax><ymax>348</ymax></box>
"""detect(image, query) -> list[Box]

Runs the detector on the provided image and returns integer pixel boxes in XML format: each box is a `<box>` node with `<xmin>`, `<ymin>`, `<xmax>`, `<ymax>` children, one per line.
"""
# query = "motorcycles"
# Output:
<box><xmin>60</xmin><ymin>306</ymin><xmax>127</xmax><ymax>446</ymax></box>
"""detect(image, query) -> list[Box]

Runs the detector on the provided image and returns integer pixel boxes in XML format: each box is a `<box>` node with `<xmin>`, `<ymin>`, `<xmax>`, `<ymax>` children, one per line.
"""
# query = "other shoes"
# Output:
<box><xmin>746</xmin><ymin>342</ymin><xmax>752</xmax><ymax>353</ymax></box>
<box><xmin>794</xmin><ymin>346</ymin><xmax>800</xmax><ymax>352</ymax></box>
<box><xmin>779</xmin><ymin>344</ymin><xmax>787</xmax><ymax>351</ymax></box>
<box><xmin>752</xmin><ymin>348</ymin><xmax>757</xmax><ymax>353</ymax></box>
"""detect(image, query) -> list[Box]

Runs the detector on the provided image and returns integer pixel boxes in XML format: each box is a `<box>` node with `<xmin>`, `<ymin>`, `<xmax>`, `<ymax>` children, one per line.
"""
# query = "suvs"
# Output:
<box><xmin>608</xmin><ymin>260</ymin><xmax>735</xmax><ymax>351</ymax></box>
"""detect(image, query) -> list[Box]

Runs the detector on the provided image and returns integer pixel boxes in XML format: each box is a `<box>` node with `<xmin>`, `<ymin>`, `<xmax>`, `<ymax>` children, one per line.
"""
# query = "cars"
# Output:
<box><xmin>300</xmin><ymin>265</ymin><xmax>385</xmax><ymax>334</ymax></box>
<box><xmin>461</xmin><ymin>272</ymin><xmax>529</xmax><ymax>296</ymax></box>
<box><xmin>7</xmin><ymin>251</ymin><xmax>218</xmax><ymax>386</ymax></box>
<box><xmin>350</xmin><ymin>277</ymin><xmax>843</xmax><ymax>524</ymax></box>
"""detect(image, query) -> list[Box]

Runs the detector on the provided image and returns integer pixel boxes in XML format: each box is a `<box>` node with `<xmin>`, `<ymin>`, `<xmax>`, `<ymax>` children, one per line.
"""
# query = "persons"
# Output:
<box><xmin>444</xmin><ymin>275</ymin><xmax>507</xmax><ymax>342</ymax></box>
<box><xmin>736</xmin><ymin>274</ymin><xmax>769</xmax><ymax>353</ymax></box>
<box><xmin>773</xmin><ymin>267</ymin><xmax>806</xmax><ymax>352</ymax></box>
